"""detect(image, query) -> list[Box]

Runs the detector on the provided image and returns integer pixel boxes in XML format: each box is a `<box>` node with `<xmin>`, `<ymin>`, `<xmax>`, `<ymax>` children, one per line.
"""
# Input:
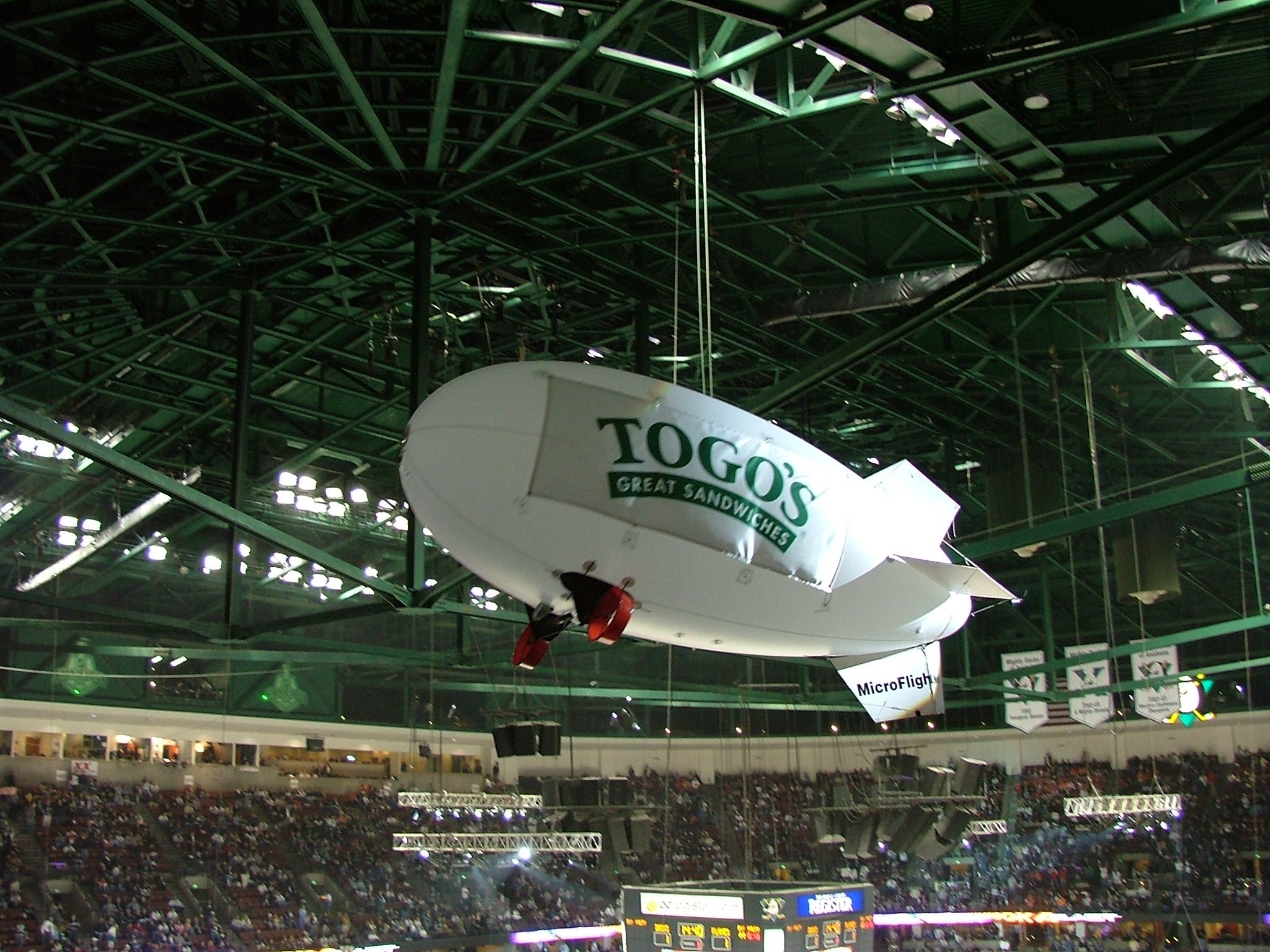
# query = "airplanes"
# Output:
<box><xmin>396</xmin><ymin>358</ymin><xmax>1024</xmax><ymax>726</ymax></box>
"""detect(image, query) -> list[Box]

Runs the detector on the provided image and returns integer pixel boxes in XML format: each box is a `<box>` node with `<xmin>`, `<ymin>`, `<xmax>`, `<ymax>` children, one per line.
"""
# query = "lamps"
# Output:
<box><xmin>887</xmin><ymin>97</ymin><xmax>906</xmax><ymax>122</ymax></box>
<box><xmin>862</xmin><ymin>76</ymin><xmax>878</xmax><ymax>105</ymax></box>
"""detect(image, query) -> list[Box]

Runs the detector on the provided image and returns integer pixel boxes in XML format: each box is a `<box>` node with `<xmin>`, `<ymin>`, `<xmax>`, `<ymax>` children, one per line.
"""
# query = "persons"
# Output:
<box><xmin>0</xmin><ymin>739</ymin><xmax>1270</xmax><ymax>952</ymax></box>
